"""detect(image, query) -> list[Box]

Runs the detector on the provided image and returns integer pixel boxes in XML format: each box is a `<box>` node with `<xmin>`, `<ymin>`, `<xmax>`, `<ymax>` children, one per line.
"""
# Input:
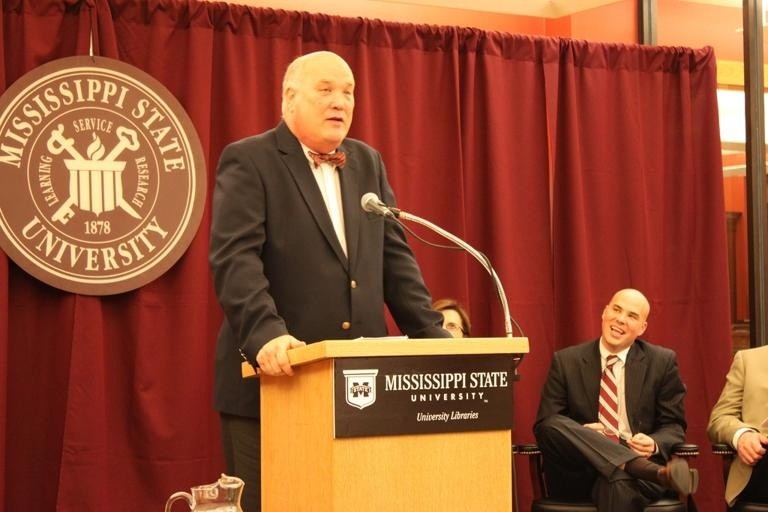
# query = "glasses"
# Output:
<box><xmin>446</xmin><ymin>323</ymin><xmax>466</xmax><ymax>334</ymax></box>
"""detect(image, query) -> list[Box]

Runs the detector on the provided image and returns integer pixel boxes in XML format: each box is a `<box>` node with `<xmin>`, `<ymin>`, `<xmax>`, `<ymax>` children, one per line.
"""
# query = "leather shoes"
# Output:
<box><xmin>665</xmin><ymin>458</ymin><xmax>700</xmax><ymax>497</ymax></box>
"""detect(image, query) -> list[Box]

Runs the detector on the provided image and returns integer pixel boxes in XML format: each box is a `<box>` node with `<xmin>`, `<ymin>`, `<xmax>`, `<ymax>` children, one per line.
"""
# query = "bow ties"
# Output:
<box><xmin>308</xmin><ymin>151</ymin><xmax>346</xmax><ymax>170</ymax></box>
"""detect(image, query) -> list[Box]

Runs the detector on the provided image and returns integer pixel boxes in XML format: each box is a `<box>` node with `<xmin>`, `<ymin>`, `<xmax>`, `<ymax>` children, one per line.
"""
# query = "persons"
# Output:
<box><xmin>706</xmin><ymin>341</ymin><xmax>768</xmax><ymax>511</ymax></box>
<box><xmin>533</xmin><ymin>288</ymin><xmax>700</xmax><ymax>512</ymax></box>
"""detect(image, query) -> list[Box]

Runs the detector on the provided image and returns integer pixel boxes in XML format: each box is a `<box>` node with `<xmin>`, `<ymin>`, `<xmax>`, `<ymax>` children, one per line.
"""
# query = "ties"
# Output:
<box><xmin>598</xmin><ymin>355</ymin><xmax>620</xmax><ymax>444</ymax></box>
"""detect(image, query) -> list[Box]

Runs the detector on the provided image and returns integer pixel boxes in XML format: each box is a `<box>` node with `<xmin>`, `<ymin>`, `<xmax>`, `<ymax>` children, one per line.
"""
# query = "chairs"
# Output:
<box><xmin>509</xmin><ymin>438</ymin><xmax>700</xmax><ymax>512</ymax></box>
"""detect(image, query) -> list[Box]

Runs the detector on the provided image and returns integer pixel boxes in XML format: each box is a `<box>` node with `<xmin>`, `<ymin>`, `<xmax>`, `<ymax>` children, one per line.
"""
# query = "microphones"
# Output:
<box><xmin>360</xmin><ymin>192</ymin><xmax>394</xmax><ymax>220</ymax></box>
